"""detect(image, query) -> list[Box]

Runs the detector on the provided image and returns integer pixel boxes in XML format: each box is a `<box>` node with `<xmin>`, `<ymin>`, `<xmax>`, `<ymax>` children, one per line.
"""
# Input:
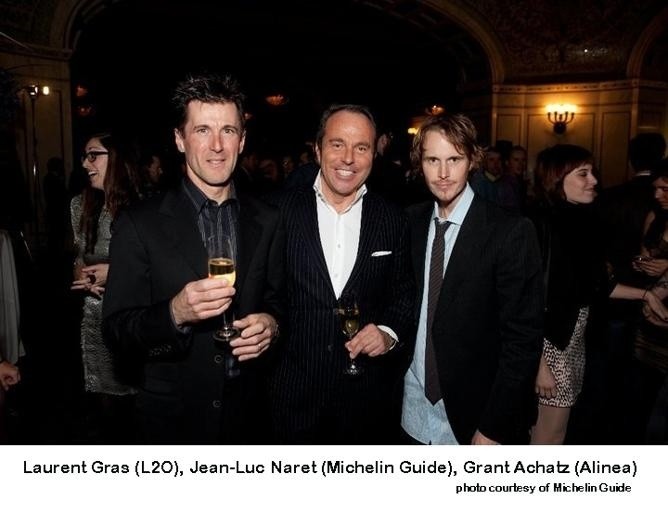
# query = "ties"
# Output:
<box><xmin>424</xmin><ymin>217</ymin><xmax>452</xmax><ymax>407</ymax></box>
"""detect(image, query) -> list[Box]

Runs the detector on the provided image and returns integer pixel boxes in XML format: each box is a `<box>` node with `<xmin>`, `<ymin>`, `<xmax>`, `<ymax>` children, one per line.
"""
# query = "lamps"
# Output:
<box><xmin>545</xmin><ymin>103</ymin><xmax>576</xmax><ymax>133</ymax></box>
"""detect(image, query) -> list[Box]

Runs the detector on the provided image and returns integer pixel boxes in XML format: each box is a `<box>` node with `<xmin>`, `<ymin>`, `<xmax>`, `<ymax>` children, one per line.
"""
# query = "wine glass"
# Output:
<box><xmin>206</xmin><ymin>233</ymin><xmax>242</xmax><ymax>341</ymax></box>
<box><xmin>335</xmin><ymin>293</ymin><xmax>367</xmax><ymax>376</ymax></box>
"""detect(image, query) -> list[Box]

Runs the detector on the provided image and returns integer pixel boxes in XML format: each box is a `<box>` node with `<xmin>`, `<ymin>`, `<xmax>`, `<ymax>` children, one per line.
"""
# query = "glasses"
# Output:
<box><xmin>80</xmin><ymin>151</ymin><xmax>108</xmax><ymax>165</ymax></box>
